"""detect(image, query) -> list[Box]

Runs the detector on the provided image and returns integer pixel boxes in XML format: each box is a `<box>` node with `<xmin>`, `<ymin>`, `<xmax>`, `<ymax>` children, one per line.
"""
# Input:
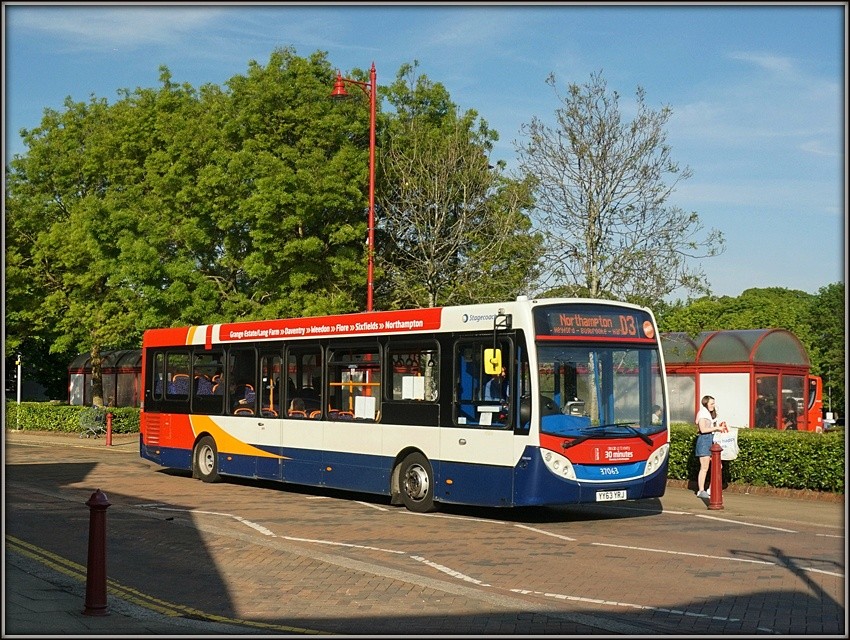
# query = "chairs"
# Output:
<box><xmin>154</xmin><ymin>372</ymin><xmax>356</xmax><ymax>419</ymax></box>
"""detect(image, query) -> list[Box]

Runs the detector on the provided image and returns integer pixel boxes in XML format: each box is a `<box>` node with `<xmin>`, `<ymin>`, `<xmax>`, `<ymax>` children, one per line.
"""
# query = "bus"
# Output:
<box><xmin>138</xmin><ymin>295</ymin><xmax>670</xmax><ymax>513</ymax></box>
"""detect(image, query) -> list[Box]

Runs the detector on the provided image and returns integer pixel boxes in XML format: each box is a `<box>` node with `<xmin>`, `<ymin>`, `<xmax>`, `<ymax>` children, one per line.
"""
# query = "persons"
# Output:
<box><xmin>651</xmin><ymin>405</ymin><xmax>661</xmax><ymax>426</ymax></box>
<box><xmin>696</xmin><ymin>395</ymin><xmax>726</xmax><ymax>499</ymax></box>
<box><xmin>782</xmin><ymin>398</ymin><xmax>799</xmax><ymax>432</ymax></box>
<box><xmin>186</xmin><ymin>365</ymin><xmax>202</xmax><ymax>376</ymax></box>
<box><xmin>754</xmin><ymin>390</ymin><xmax>778</xmax><ymax>431</ymax></box>
<box><xmin>484</xmin><ymin>366</ymin><xmax>510</xmax><ymax>403</ymax></box>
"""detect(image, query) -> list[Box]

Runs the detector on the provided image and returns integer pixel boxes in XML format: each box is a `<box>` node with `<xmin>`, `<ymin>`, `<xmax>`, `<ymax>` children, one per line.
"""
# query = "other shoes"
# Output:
<box><xmin>697</xmin><ymin>490</ymin><xmax>711</xmax><ymax>499</ymax></box>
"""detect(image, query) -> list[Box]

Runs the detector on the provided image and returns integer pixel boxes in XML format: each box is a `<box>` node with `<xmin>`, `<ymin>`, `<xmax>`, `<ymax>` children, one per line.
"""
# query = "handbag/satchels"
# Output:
<box><xmin>714</xmin><ymin>423</ymin><xmax>741</xmax><ymax>461</ymax></box>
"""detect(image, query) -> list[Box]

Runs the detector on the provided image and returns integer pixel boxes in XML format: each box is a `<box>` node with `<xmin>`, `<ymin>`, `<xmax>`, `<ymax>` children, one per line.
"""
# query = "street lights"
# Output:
<box><xmin>327</xmin><ymin>61</ymin><xmax>376</xmax><ymax>396</ymax></box>
